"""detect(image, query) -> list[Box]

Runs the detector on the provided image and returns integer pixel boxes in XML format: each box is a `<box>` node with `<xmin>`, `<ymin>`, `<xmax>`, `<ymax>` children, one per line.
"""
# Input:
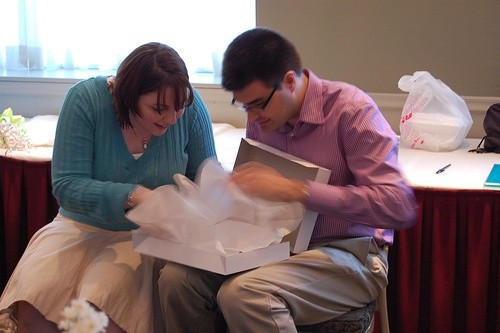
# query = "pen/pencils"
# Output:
<box><xmin>436</xmin><ymin>164</ymin><xmax>451</xmax><ymax>174</ymax></box>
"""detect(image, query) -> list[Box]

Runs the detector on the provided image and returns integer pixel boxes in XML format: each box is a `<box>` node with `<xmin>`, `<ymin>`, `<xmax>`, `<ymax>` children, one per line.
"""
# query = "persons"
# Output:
<box><xmin>158</xmin><ymin>28</ymin><xmax>414</xmax><ymax>333</ymax></box>
<box><xmin>0</xmin><ymin>42</ymin><xmax>220</xmax><ymax>333</ymax></box>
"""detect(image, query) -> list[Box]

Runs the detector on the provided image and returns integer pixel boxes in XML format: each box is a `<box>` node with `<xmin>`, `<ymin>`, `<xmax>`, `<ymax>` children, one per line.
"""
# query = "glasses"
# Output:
<box><xmin>231</xmin><ymin>76</ymin><xmax>281</xmax><ymax>112</ymax></box>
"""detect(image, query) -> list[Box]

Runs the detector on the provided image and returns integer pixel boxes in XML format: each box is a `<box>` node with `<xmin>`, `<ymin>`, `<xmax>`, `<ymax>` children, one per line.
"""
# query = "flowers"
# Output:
<box><xmin>0</xmin><ymin>107</ymin><xmax>36</xmax><ymax>153</ymax></box>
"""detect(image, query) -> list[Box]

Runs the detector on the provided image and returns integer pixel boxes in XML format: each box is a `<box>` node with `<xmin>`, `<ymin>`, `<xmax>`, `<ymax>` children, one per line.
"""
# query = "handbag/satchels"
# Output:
<box><xmin>397</xmin><ymin>70</ymin><xmax>473</xmax><ymax>151</ymax></box>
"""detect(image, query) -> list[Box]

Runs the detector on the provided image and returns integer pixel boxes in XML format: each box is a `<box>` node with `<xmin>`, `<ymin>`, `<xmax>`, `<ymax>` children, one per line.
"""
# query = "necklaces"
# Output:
<box><xmin>143</xmin><ymin>137</ymin><xmax>147</xmax><ymax>149</ymax></box>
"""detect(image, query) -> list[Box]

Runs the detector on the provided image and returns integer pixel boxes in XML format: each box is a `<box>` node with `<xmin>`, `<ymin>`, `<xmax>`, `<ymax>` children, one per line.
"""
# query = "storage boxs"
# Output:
<box><xmin>134</xmin><ymin>138</ymin><xmax>332</xmax><ymax>277</ymax></box>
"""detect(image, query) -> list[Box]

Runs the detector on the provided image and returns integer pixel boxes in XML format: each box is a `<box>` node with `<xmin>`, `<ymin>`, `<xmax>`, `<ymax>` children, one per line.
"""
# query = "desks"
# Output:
<box><xmin>0</xmin><ymin>122</ymin><xmax>500</xmax><ymax>333</ymax></box>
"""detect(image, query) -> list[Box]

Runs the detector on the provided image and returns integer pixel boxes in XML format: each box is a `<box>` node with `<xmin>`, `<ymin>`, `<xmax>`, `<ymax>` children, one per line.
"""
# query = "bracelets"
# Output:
<box><xmin>126</xmin><ymin>184</ymin><xmax>142</xmax><ymax>208</ymax></box>
